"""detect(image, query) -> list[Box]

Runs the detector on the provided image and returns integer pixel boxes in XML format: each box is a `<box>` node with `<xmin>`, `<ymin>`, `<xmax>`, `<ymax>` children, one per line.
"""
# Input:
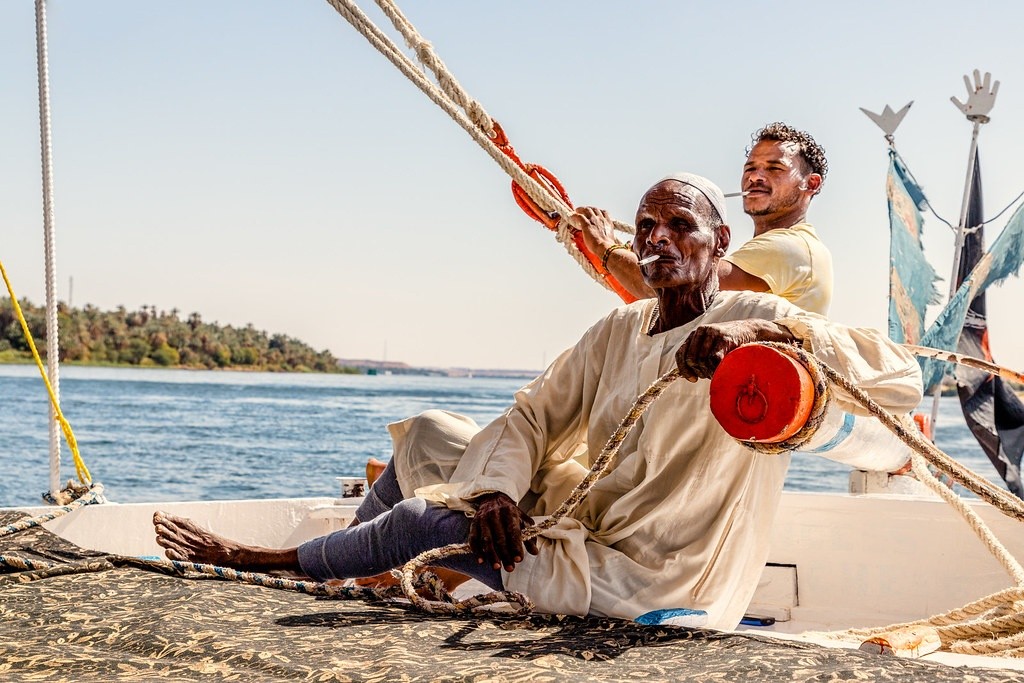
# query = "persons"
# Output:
<box><xmin>567</xmin><ymin>123</ymin><xmax>832</xmax><ymax>315</ymax></box>
<box><xmin>153</xmin><ymin>172</ymin><xmax>924</xmax><ymax>633</ymax></box>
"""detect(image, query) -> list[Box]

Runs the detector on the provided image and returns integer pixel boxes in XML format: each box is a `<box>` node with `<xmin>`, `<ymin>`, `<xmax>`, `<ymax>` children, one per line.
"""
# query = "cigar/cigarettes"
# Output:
<box><xmin>724</xmin><ymin>192</ymin><xmax>753</xmax><ymax>197</ymax></box>
<box><xmin>636</xmin><ymin>253</ymin><xmax>660</xmax><ymax>268</ymax></box>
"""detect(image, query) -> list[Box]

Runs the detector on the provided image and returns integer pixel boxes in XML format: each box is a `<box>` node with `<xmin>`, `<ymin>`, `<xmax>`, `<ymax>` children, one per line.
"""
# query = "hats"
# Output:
<box><xmin>635</xmin><ymin>170</ymin><xmax>731</xmax><ymax>224</ymax></box>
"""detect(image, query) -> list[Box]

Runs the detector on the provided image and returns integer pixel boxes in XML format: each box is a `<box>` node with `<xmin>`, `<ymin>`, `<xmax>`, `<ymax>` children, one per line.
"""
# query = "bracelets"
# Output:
<box><xmin>600</xmin><ymin>242</ymin><xmax>628</xmax><ymax>272</ymax></box>
